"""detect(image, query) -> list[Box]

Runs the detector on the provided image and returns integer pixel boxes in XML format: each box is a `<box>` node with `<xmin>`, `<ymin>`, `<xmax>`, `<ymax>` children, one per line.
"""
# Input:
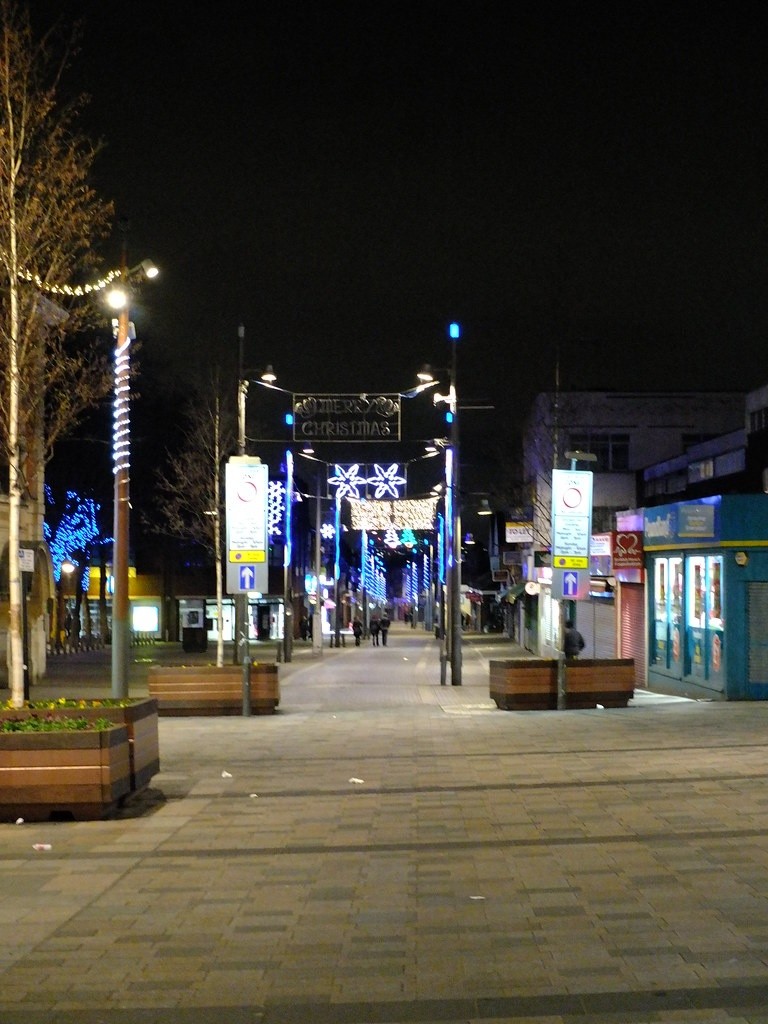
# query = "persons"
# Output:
<box><xmin>353</xmin><ymin>617</ymin><xmax>362</xmax><ymax>646</ymax></box>
<box><xmin>403</xmin><ymin>612</ymin><xmax>413</xmax><ymax>626</ymax></box>
<box><xmin>299</xmin><ymin>615</ymin><xmax>308</xmax><ymax>641</ymax></box>
<box><xmin>564</xmin><ymin>620</ymin><xmax>585</xmax><ymax>660</ymax></box>
<box><xmin>307</xmin><ymin>615</ymin><xmax>312</xmax><ymax>638</ymax></box>
<box><xmin>369</xmin><ymin>613</ymin><xmax>390</xmax><ymax>646</ymax></box>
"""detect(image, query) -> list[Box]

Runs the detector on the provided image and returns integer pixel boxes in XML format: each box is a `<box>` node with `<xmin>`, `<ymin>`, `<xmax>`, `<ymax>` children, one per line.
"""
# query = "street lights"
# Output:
<box><xmin>417</xmin><ymin>363</ymin><xmax>463</xmax><ymax>688</ymax></box>
<box><xmin>555</xmin><ymin>450</ymin><xmax>598</xmax><ymax>709</ymax></box>
<box><xmin>98</xmin><ymin>258</ymin><xmax>160</xmax><ymax>703</ymax></box>
<box><xmin>232</xmin><ymin>364</ymin><xmax>279</xmax><ymax>666</ymax></box>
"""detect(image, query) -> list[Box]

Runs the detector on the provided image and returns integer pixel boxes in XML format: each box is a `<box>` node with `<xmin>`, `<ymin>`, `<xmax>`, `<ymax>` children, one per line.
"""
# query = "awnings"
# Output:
<box><xmin>505</xmin><ymin>584</ymin><xmax>526</xmax><ymax>604</ymax></box>
<box><xmin>495</xmin><ymin>592</ymin><xmax>507</xmax><ymax>604</ymax></box>
